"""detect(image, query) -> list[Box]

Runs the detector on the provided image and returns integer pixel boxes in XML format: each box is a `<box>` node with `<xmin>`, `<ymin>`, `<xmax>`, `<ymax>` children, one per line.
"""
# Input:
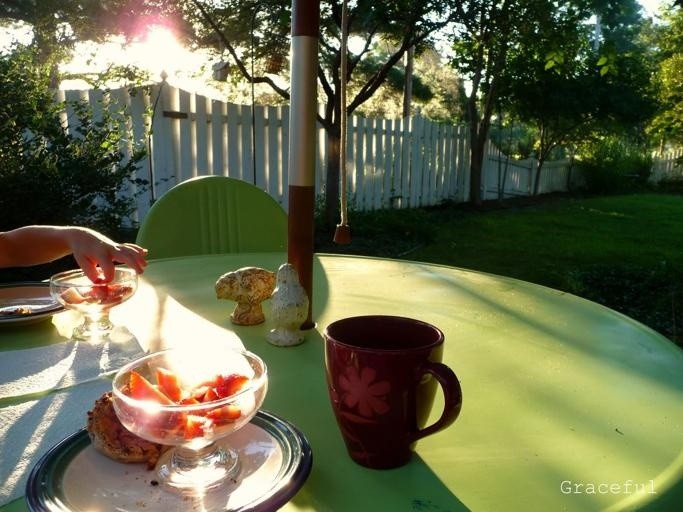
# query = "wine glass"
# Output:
<box><xmin>111</xmin><ymin>344</ymin><xmax>270</xmax><ymax>495</ymax></box>
<box><xmin>49</xmin><ymin>266</ymin><xmax>137</xmax><ymax>342</ymax></box>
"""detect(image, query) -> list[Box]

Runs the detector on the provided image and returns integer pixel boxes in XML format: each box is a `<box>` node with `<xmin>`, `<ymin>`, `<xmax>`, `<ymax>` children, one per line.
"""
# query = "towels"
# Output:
<box><xmin>0</xmin><ymin>252</ymin><xmax>682</xmax><ymax>512</ymax></box>
<box><xmin>0</xmin><ymin>326</ymin><xmax>149</xmax><ymax>402</ymax></box>
<box><xmin>0</xmin><ymin>379</ymin><xmax>120</xmax><ymax>506</ymax></box>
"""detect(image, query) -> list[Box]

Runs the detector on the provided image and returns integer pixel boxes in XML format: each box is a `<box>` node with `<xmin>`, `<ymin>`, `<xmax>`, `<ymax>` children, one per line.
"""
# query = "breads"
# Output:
<box><xmin>86</xmin><ymin>389</ymin><xmax>159</xmax><ymax>470</ymax></box>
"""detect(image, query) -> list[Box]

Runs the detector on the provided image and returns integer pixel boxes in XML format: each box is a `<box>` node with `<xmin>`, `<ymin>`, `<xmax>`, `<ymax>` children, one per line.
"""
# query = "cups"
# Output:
<box><xmin>323</xmin><ymin>314</ymin><xmax>462</xmax><ymax>471</ymax></box>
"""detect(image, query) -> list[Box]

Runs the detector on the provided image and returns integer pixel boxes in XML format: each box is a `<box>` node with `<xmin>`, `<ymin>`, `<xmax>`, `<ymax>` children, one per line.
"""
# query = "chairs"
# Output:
<box><xmin>134</xmin><ymin>173</ymin><xmax>288</xmax><ymax>260</ymax></box>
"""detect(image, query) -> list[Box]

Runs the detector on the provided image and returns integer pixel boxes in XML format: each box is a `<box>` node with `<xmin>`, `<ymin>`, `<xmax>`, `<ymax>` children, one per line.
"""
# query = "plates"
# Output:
<box><xmin>0</xmin><ymin>278</ymin><xmax>78</xmax><ymax>328</ymax></box>
<box><xmin>24</xmin><ymin>406</ymin><xmax>311</xmax><ymax>512</ymax></box>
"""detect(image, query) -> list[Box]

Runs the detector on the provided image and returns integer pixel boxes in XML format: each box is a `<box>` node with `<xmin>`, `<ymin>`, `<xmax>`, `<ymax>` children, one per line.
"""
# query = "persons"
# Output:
<box><xmin>0</xmin><ymin>224</ymin><xmax>150</xmax><ymax>284</ymax></box>
